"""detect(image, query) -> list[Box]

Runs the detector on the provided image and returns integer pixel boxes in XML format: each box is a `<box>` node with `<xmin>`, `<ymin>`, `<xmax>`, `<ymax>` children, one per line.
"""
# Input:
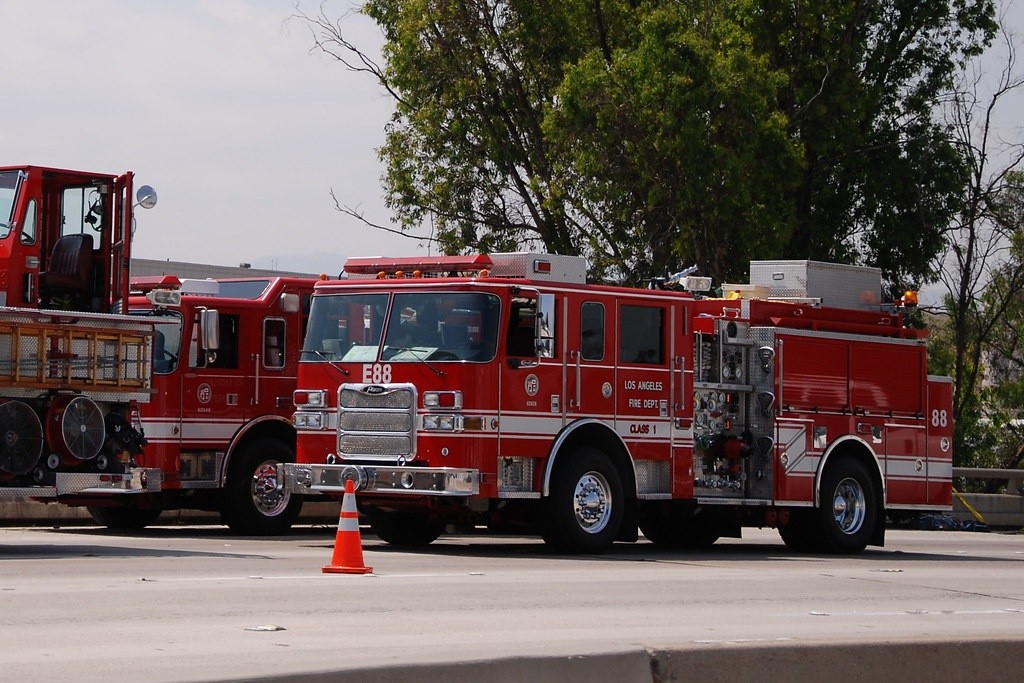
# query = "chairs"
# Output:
<box><xmin>152</xmin><ymin>331</ymin><xmax>165</xmax><ymax>360</ymax></box>
<box><xmin>38</xmin><ymin>234</ymin><xmax>93</xmax><ymax>309</ymax></box>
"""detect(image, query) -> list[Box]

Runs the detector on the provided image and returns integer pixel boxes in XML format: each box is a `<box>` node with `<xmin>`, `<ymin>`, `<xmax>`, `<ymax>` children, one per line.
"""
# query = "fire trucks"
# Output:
<box><xmin>275</xmin><ymin>253</ymin><xmax>954</xmax><ymax>559</ymax></box>
<box><xmin>0</xmin><ymin>163</ymin><xmax>534</xmax><ymax>540</ymax></box>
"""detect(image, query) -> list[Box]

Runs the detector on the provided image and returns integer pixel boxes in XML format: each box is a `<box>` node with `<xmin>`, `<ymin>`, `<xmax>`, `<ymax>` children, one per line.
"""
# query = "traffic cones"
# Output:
<box><xmin>320</xmin><ymin>478</ymin><xmax>375</xmax><ymax>573</ymax></box>
